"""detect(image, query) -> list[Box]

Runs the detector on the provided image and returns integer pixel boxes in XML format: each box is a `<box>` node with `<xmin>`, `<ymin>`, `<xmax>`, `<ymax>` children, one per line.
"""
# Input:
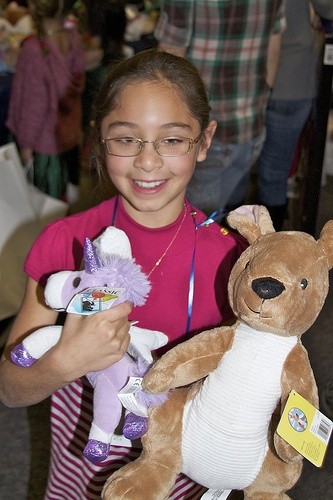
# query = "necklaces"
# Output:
<box><xmin>110</xmin><ymin>194</ymin><xmax>188</xmax><ymax>280</ymax></box>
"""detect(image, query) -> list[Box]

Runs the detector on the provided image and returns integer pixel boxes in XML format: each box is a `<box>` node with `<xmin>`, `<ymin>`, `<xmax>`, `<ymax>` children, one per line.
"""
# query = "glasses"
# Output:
<box><xmin>98</xmin><ymin>132</ymin><xmax>203</xmax><ymax>157</ymax></box>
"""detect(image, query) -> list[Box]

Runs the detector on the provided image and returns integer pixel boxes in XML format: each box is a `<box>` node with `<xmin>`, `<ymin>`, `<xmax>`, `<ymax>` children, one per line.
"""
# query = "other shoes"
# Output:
<box><xmin>253</xmin><ymin>198</ymin><xmax>289</xmax><ymax>232</ymax></box>
<box><xmin>222</xmin><ymin>200</ymin><xmax>244</xmax><ymax>231</ymax></box>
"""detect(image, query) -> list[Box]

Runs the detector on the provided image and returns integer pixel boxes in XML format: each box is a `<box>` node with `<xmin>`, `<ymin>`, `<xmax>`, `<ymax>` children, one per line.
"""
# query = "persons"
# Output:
<box><xmin>5</xmin><ymin>1</ymin><xmax>88</xmax><ymax>200</ymax></box>
<box><xmin>155</xmin><ymin>0</ymin><xmax>284</xmax><ymax>222</ymax></box>
<box><xmin>255</xmin><ymin>0</ymin><xmax>332</xmax><ymax>235</ymax></box>
<box><xmin>0</xmin><ymin>50</ymin><xmax>249</xmax><ymax>500</ymax></box>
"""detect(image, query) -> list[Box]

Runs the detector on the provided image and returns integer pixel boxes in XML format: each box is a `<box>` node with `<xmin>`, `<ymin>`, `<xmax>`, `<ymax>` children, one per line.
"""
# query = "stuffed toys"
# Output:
<box><xmin>98</xmin><ymin>202</ymin><xmax>333</xmax><ymax>500</ymax></box>
<box><xmin>10</xmin><ymin>227</ymin><xmax>171</xmax><ymax>462</ymax></box>
